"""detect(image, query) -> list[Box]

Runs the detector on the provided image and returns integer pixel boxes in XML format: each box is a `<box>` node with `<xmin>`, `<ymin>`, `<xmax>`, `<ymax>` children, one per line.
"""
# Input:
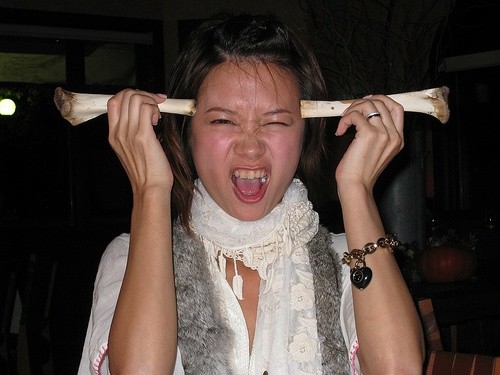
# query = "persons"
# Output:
<box><xmin>75</xmin><ymin>14</ymin><xmax>418</xmax><ymax>374</ymax></box>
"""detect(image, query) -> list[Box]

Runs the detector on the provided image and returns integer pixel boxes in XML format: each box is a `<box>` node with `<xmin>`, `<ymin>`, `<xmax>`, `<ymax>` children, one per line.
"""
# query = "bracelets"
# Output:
<box><xmin>343</xmin><ymin>233</ymin><xmax>401</xmax><ymax>291</ymax></box>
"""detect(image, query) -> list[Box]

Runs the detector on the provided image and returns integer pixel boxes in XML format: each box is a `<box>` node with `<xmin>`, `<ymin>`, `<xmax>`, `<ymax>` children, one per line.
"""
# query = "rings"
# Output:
<box><xmin>365</xmin><ymin>111</ymin><xmax>380</xmax><ymax>118</ymax></box>
<box><xmin>366</xmin><ymin>114</ymin><xmax>382</xmax><ymax>120</ymax></box>
